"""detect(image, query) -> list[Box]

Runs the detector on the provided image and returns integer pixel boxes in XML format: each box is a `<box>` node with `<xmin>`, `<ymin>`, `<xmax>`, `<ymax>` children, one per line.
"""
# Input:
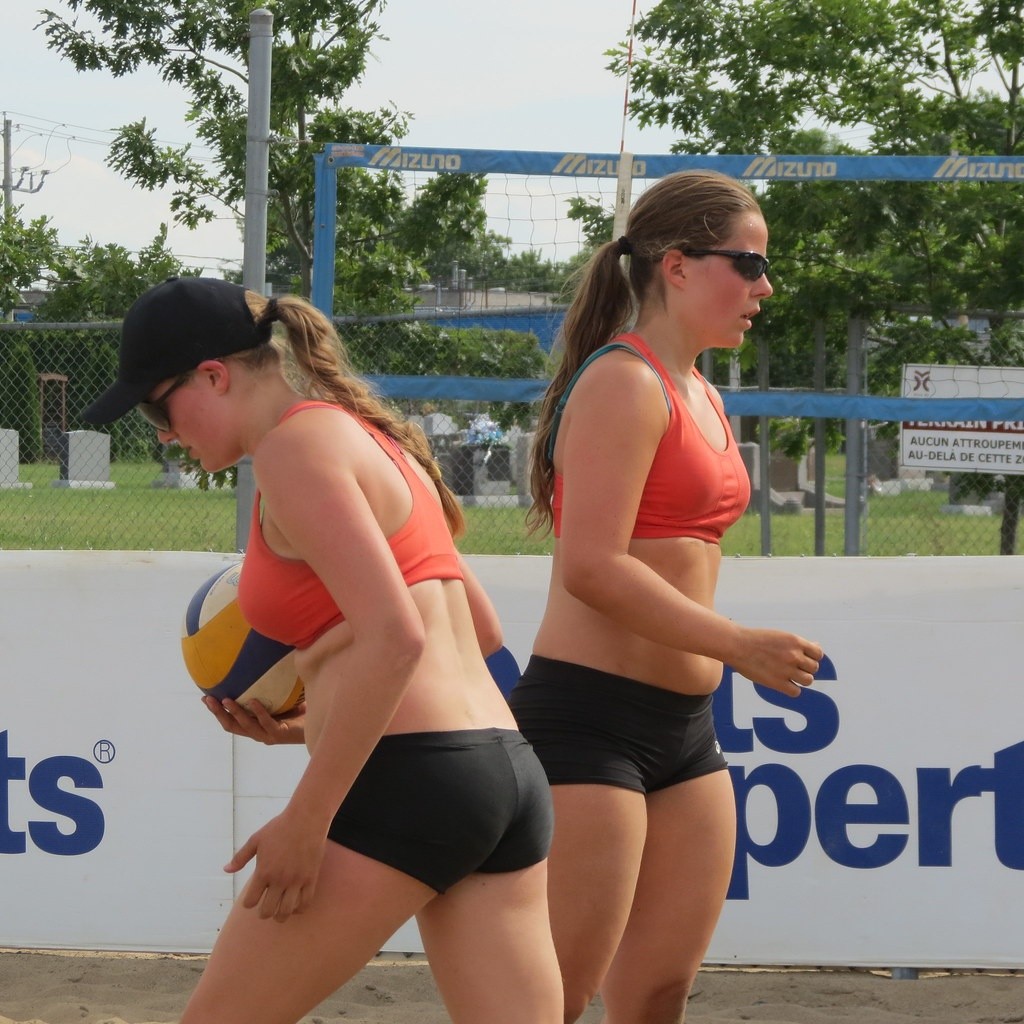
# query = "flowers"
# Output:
<box><xmin>465</xmin><ymin>410</ymin><xmax>504</xmax><ymax>450</ymax></box>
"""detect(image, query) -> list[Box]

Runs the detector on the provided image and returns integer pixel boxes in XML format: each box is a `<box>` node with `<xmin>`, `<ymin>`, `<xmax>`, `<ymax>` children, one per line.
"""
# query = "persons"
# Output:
<box><xmin>79</xmin><ymin>276</ymin><xmax>565</xmax><ymax>1024</ymax></box>
<box><xmin>511</xmin><ymin>169</ymin><xmax>822</xmax><ymax>1024</ymax></box>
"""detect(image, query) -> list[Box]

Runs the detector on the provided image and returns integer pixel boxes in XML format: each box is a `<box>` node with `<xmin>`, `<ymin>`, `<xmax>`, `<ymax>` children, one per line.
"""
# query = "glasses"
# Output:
<box><xmin>652</xmin><ymin>249</ymin><xmax>772</xmax><ymax>282</ymax></box>
<box><xmin>136</xmin><ymin>372</ymin><xmax>188</xmax><ymax>432</ymax></box>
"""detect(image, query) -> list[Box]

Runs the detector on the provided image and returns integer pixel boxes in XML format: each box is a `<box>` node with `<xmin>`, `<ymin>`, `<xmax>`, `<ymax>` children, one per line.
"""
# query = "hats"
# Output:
<box><xmin>81</xmin><ymin>276</ymin><xmax>273</xmax><ymax>426</ymax></box>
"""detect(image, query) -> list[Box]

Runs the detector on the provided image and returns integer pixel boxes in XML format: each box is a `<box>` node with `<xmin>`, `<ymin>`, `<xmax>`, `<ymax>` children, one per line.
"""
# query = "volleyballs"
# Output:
<box><xmin>180</xmin><ymin>555</ymin><xmax>306</xmax><ymax>717</ymax></box>
